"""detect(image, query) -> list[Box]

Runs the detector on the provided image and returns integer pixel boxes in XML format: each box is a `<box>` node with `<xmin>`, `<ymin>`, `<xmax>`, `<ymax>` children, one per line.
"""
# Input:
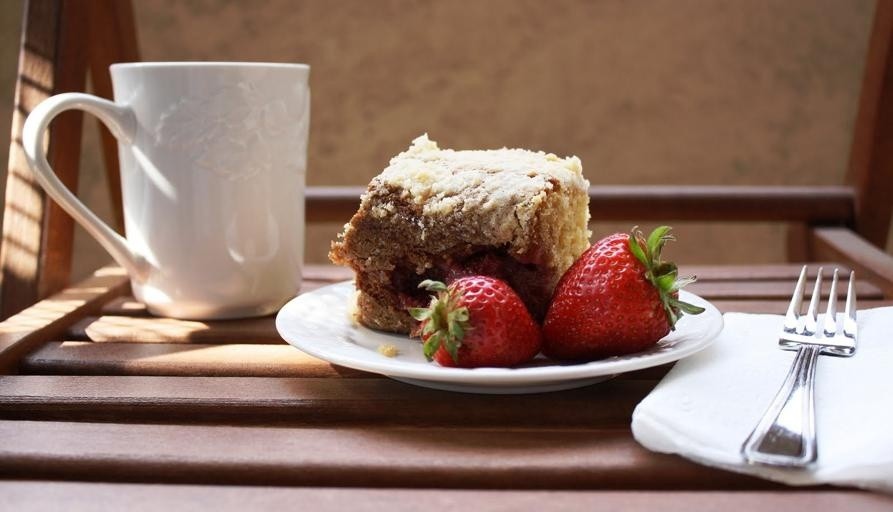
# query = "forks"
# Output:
<box><xmin>741</xmin><ymin>264</ymin><xmax>861</xmax><ymax>465</ymax></box>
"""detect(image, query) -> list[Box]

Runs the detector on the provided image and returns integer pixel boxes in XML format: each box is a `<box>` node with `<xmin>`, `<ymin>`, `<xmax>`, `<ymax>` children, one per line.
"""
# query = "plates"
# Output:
<box><xmin>276</xmin><ymin>279</ymin><xmax>725</xmax><ymax>395</ymax></box>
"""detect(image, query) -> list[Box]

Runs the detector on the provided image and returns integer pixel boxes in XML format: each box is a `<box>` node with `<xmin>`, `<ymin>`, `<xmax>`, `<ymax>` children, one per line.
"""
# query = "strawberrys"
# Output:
<box><xmin>541</xmin><ymin>233</ymin><xmax>679</xmax><ymax>358</ymax></box>
<box><xmin>418</xmin><ymin>275</ymin><xmax>541</xmax><ymax>367</ymax></box>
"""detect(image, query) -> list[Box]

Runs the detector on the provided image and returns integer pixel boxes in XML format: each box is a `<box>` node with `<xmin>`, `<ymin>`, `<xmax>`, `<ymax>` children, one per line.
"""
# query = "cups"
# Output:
<box><xmin>19</xmin><ymin>61</ymin><xmax>314</xmax><ymax>320</ymax></box>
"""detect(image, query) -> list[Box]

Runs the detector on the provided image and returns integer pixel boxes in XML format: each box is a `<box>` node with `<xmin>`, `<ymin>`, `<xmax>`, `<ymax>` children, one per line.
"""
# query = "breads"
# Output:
<box><xmin>328</xmin><ymin>133</ymin><xmax>593</xmax><ymax>336</ymax></box>
